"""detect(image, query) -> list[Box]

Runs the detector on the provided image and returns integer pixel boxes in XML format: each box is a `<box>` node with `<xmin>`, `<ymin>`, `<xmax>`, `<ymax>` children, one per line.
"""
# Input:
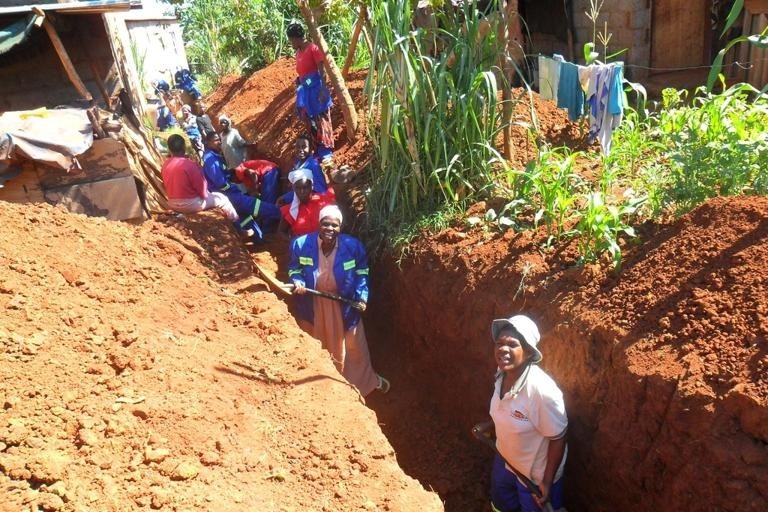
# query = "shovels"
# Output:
<box><xmin>252</xmin><ymin>260</ymin><xmax>363</xmax><ymax>312</ymax></box>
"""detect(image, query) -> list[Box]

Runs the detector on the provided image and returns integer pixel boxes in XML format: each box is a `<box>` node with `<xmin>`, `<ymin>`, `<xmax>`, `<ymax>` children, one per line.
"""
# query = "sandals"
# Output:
<box><xmin>374</xmin><ymin>373</ymin><xmax>391</xmax><ymax>395</ymax></box>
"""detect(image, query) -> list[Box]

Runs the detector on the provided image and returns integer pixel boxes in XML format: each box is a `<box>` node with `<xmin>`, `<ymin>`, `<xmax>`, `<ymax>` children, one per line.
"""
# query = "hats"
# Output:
<box><xmin>490</xmin><ymin>313</ymin><xmax>543</xmax><ymax>365</ymax></box>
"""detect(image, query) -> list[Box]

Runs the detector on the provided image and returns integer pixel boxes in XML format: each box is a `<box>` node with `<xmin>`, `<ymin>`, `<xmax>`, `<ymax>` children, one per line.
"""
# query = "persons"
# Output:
<box><xmin>471</xmin><ymin>313</ymin><xmax>572</xmax><ymax>512</ymax></box>
<box><xmin>202</xmin><ymin>132</ymin><xmax>281</xmax><ymax>241</ymax></box>
<box><xmin>161</xmin><ymin>133</ymin><xmax>254</xmax><ymax>240</ymax></box>
<box><xmin>153</xmin><ymin>63</ymin><xmax>215</xmax><ymax>157</ymax></box>
<box><xmin>286</xmin><ymin>23</ymin><xmax>335</xmax><ymax>158</ymax></box>
<box><xmin>272</xmin><ymin>133</ymin><xmax>327</xmax><ymax>210</ymax></box>
<box><xmin>228</xmin><ymin>159</ymin><xmax>282</xmax><ymax>205</ymax></box>
<box><xmin>275</xmin><ymin>167</ymin><xmax>337</xmax><ymax>241</ymax></box>
<box><xmin>217</xmin><ymin>115</ymin><xmax>256</xmax><ymax>167</ymax></box>
<box><xmin>287</xmin><ymin>205</ymin><xmax>393</xmax><ymax>400</ymax></box>
